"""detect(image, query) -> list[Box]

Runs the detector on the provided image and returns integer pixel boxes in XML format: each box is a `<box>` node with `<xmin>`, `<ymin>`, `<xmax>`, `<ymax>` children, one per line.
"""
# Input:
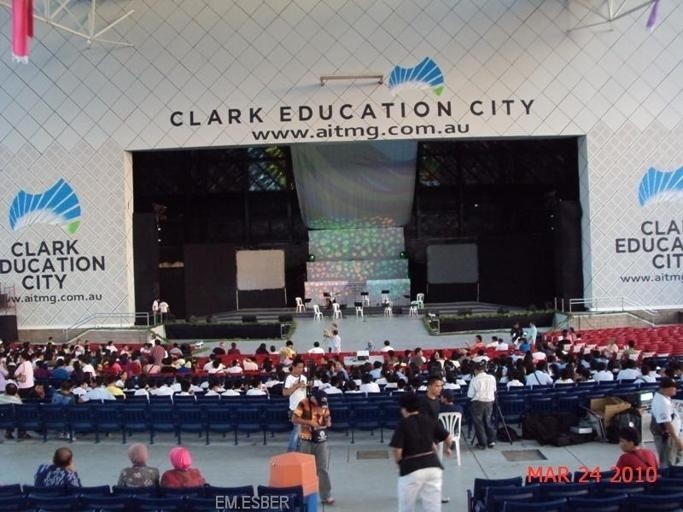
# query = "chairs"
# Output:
<box><xmin>0</xmin><ymin>324</ymin><xmax>683</xmax><ymax>511</ymax></box>
<box><xmin>296</xmin><ymin>291</ymin><xmax>424</xmax><ymax>321</ymax></box>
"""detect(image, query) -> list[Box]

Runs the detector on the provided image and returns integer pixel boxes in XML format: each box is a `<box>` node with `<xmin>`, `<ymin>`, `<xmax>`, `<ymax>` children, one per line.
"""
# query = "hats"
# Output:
<box><xmin>312</xmin><ymin>390</ymin><xmax>328</xmax><ymax>407</ymax></box>
<box><xmin>659</xmin><ymin>377</ymin><xmax>682</xmax><ymax>388</ymax></box>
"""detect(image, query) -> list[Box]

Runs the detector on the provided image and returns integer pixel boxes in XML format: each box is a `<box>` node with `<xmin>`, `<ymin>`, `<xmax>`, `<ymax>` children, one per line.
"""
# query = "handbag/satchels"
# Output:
<box><xmin>310</xmin><ymin>423</ymin><xmax>329</xmax><ymax>443</ymax></box>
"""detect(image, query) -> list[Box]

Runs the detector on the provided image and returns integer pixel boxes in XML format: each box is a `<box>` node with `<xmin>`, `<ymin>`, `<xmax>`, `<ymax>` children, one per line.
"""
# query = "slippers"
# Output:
<box><xmin>476</xmin><ymin>443</ymin><xmax>486</xmax><ymax>449</ymax></box>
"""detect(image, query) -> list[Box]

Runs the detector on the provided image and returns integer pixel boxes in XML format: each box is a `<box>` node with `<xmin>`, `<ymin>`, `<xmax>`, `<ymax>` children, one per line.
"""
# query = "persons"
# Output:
<box><xmin>151</xmin><ymin>297</ymin><xmax>161</xmax><ymax>324</ymax></box>
<box><xmin>158</xmin><ymin>299</ymin><xmax>169</xmax><ymax>323</ymax></box>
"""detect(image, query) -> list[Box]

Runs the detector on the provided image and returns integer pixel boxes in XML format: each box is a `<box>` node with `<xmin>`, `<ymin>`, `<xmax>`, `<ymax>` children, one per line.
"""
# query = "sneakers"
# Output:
<box><xmin>321</xmin><ymin>497</ymin><xmax>335</xmax><ymax>504</ymax></box>
